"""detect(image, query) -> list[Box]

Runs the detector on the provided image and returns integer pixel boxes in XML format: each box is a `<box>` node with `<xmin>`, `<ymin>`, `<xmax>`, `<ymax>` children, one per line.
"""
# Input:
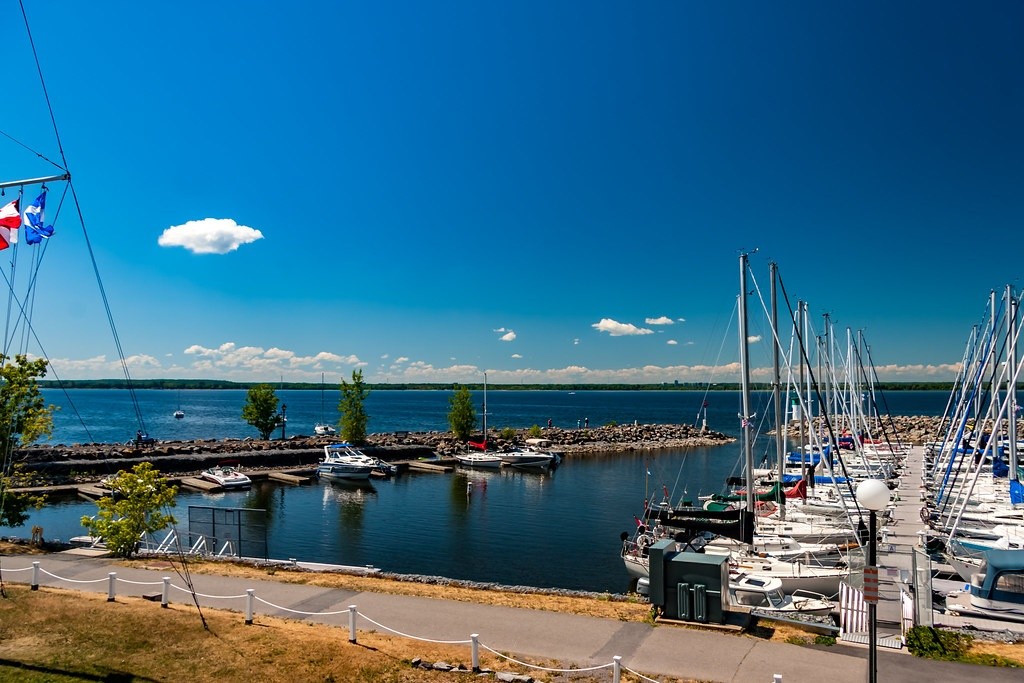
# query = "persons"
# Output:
<box><xmin>576</xmin><ymin>417</ymin><xmax>589</xmax><ymax>429</ymax></box>
<box><xmin>637</xmin><ymin>526</ymin><xmax>652</xmax><ymax>558</ymax></box>
<box><xmin>547</xmin><ymin>418</ymin><xmax>552</xmax><ymax>428</ymax></box>
<box><xmin>137</xmin><ymin>430</ymin><xmax>142</xmax><ymax>440</ymax></box>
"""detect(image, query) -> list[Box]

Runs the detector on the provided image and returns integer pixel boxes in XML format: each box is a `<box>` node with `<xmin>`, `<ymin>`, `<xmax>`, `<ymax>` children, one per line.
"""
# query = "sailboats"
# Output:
<box><xmin>269</xmin><ymin>374</ymin><xmax>287</xmax><ymax>427</ymax></box>
<box><xmin>173</xmin><ymin>389</ymin><xmax>184</xmax><ymax>418</ymax></box>
<box><xmin>314</xmin><ymin>373</ymin><xmax>335</xmax><ymax>436</ymax></box>
<box><xmin>456</xmin><ymin>371</ymin><xmax>502</xmax><ymax>467</ymax></box>
<box><xmin>621</xmin><ymin>245</ymin><xmax>914</xmax><ymax>637</ymax></box>
<box><xmin>918</xmin><ymin>283</ymin><xmax>1024</xmax><ymax>622</ymax></box>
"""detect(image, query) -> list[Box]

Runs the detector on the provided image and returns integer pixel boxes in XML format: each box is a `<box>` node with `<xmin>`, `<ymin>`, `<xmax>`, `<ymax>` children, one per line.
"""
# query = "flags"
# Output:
<box><xmin>23</xmin><ymin>189</ymin><xmax>56</xmax><ymax>245</ymax></box>
<box><xmin>741</xmin><ymin>417</ymin><xmax>747</xmax><ymax>428</ymax></box>
<box><xmin>0</xmin><ymin>196</ymin><xmax>21</xmax><ymax>251</ymax></box>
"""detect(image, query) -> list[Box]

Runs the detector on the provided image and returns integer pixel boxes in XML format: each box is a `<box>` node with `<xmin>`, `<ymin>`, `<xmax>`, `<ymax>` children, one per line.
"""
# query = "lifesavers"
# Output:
<box><xmin>637</xmin><ymin>534</ymin><xmax>651</xmax><ymax>549</ymax></box>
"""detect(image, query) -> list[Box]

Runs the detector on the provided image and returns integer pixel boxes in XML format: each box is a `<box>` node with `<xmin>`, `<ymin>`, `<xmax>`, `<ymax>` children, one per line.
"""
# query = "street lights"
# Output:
<box><xmin>856</xmin><ymin>479</ymin><xmax>890</xmax><ymax>683</ymax></box>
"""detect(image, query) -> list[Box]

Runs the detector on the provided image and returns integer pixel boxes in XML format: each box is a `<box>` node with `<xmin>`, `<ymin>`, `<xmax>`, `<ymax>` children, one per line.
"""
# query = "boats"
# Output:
<box><xmin>100</xmin><ymin>476</ymin><xmax>157</xmax><ymax>497</ymax></box>
<box><xmin>68</xmin><ymin>534</ymin><xmax>103</xmax><ymax>547</ymax></box>
<box><xmin>524</xmin><ymin>439</ymin><xmax>561</xmax><ymax>465</ymax></box>
<box><xmin>371</xmin><ymin>456</ymin><xmax>398</xmax><ymax>475</ymax></box>
<box><xmin>316</xmin><ymin>442</ymin><xmax>375</xmax><ymax>481</ymax></box>
<box><xmin>201</xmin><ymin>464</ymin><xmax>252</xmax><ymax>490</ymax></box>
<box><xmin>491</xmin><ymin>443</ymin><xmax>553</xmax><ymax>469</ymax></box>
